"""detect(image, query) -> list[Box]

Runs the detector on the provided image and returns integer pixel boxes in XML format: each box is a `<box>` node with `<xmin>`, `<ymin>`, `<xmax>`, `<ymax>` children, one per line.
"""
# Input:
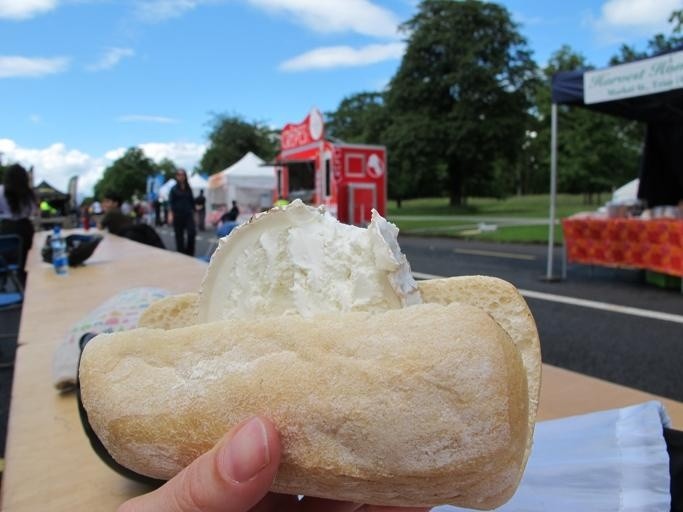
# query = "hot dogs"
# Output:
<box><xmin>78</xmin><ymin>198</ymin><xmax>542</xmax><ymax>510</ymax></box>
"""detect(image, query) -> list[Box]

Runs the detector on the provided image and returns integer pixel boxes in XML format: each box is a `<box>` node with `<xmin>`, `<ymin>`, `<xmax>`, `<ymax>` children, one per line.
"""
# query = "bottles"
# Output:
<box><xmin>50</xmin><ymin>226</ymin><xmax>70</xmax><ymax>276</ymax></box>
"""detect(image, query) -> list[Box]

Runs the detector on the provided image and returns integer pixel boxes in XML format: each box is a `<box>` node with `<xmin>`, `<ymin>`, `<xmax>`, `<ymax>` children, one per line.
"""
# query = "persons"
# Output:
<box><xmin>0</xmin><ymin>163</ymin><xmax>241</xmax><ymax>294</ymax></box>
<box><xmin>113</xmin><ymin>415</ymin><xmax>433</xmax><ymax>511</ymax></box>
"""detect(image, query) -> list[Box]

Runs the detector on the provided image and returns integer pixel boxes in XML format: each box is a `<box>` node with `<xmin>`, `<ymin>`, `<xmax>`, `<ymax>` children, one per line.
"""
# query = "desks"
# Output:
<box><xmin>559</xmin><ymin>212</ymin><xmax>682</xmax><ymax>281</ymax></box>
<box><xmin>3</xmin><ymin>228</ymin><xmax>683</xmax><ymax>506</ymax></box>
